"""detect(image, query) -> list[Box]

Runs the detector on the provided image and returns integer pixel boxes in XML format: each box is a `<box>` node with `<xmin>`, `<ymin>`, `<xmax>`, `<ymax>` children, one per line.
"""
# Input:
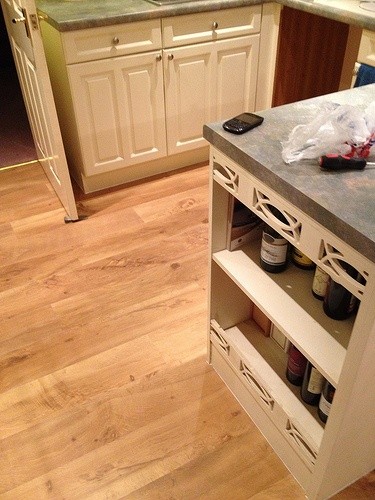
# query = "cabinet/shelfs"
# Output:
<box><xmin>35</xmin><ymin>0</ymin><xmax>375</xmax><ymax>500</ymax></box>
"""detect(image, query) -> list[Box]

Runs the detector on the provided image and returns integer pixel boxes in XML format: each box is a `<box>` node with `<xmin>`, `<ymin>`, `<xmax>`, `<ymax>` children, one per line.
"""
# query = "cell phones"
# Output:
<box><xmin>223</xmin><ymin>113</ymin><xmax>264</xmax><ymax>135</ymax></box>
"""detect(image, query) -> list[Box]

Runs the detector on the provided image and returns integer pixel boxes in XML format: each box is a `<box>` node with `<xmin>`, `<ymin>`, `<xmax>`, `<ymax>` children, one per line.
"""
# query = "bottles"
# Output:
<box><xmin>286</xmin><ymin>341</ymin><xmax>335</xmax><ymax>423</ymax></box>
<box><xmin>259</xmin><ymin>206</ymin><xmax>366</xmax><ymax>320</ymax></box>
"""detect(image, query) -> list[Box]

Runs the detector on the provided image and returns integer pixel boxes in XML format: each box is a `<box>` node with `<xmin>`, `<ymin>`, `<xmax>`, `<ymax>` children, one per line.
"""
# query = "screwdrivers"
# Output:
<box><xmin>318</xmin><ymin>154</ymin><xmax>375</xmax><ymax>172</ymax></box>
